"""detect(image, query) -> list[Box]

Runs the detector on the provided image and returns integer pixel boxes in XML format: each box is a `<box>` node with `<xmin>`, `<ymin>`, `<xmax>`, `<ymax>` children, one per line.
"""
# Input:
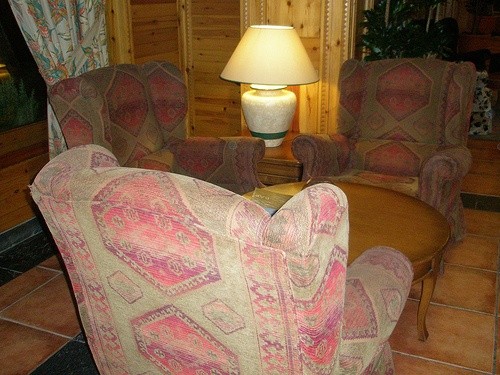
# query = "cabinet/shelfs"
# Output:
<box><xmin>461</xmin><ymin>33</ymin><xmax>500</xmax><ymax>56</ymax></box>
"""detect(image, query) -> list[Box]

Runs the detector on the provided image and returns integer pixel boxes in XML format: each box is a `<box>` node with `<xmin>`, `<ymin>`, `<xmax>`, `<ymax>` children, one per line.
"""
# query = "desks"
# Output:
<box><xmin>233</xmin><ymin>126</ymin><xmax>307</xmax><ymax>185</ymax></box>
<box><xmin>242</xmin><ymin>178</ymin><xmax>451</xmax><ymax>343</ymax></box>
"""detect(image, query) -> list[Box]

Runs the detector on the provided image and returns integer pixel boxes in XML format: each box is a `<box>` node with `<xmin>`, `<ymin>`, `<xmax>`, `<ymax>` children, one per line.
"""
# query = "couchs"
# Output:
<box><xmin>291</xmin><ymin>58</ymin><xmax>478</xmax><ymax>272</ymax></box>
<box><xmin>29</xmin><ymin>145</ymin><xmax>414</xmax><ymax>375</ymax></box>
<box><xmin>49</xmin><ymin>60</ymin><xmax>265</xmax><ymax>196</ymax></box>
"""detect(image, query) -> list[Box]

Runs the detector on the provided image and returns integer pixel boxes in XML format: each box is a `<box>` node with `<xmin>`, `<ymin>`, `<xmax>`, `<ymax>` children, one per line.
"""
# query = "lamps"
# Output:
<box><xmin>220</xmin><ymin>23</ymin><xmax>320</xmax><ymax>148</ymax></box>
